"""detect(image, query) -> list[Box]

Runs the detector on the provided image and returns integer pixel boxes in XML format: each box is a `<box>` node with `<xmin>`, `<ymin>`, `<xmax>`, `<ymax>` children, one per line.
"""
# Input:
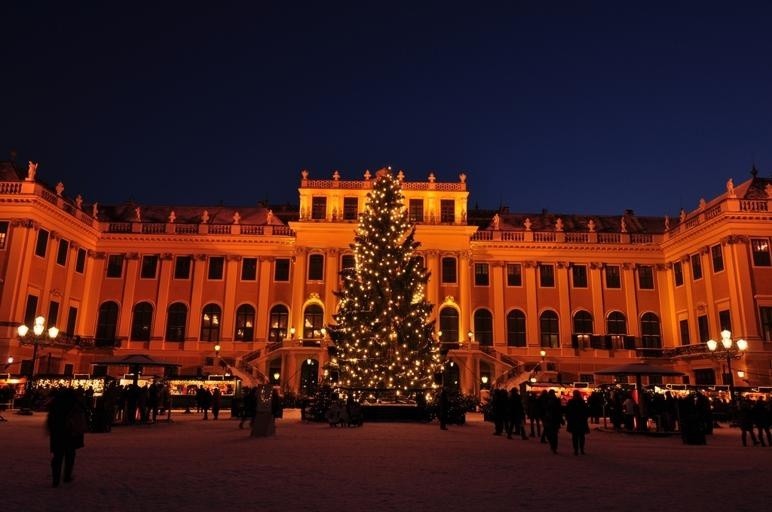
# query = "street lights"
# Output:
<box><xmin>16</xmin><ymin>315</ymin><xmax>60</xmax><ymax>415</ymax></box>
<box><xmin>706</xmin><ymin>329</ymin><xmax>749</xmax><ymax>428</ymax></box>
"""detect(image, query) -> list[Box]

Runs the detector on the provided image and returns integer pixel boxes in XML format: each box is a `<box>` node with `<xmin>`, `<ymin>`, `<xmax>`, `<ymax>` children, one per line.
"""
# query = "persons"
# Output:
<box><xmin>492</xmin><ymin>213</ymin><xmax>500</xmax><ymax>231</ymax></box>
<box><xmin>266</xmin><ymin>209</ymin><xmax>273</xmax><ymax>224</ymax></box>
<box><xmin>416</xmin><ymin>390</ymin><xmax>427</xmax><ymax>422</ymax></box>
<box><xmin>461</xmin><ymin>209</ymin><xmax>466</xmax><ymax>224</ymax></box>
<box><xmin>93</xmin><ymin>202</ymin><xmax>99</xmax><ymax>219</ymax></box>
<box><xmin>236</xmin><ymin>386</ymin><xmax>257</xmax><ymax>429</ymax></box>
<box><xmin>25</xmin><ymin>160</ymin><xmax>38</xmax><ymax>180</ymax></box>
<box><xmin>339</xmin><ymin>401</ymin><xmax>348</xmax><ymax>428</ymax></box>
<box><xmin>54</xmin><ymin>181</ymin><xmax>64</xmax><ymax>197</ymax></box>
<box><xmin>76</xmin><ymin>194</ymin><xmax>83</xmax><ymax>208</ymax></box>
<box><xmin>351</xmin><ymin>402</ymin><xmax>365</xmax><ymax>426</ymax></box>
<box><xmin>0</xmin><ymin>376</ymin><xmax>234</xmax><ymax>434</ymax></box>
<box><xmin>332</xmin><ymin>207</ymin><xmax>338</xmax><ymax>221</ymax></box>
<box><xmin>345</xmin><ymin>392</ymin><xmax>356</xmax><ymax>428</ymax></box>
<box><xmin>42</xmin><ymin>385</ymin><xmax>85</xmax><ymax>487</ymax></box>
<box><xmin>325</xmin><ymin>399</ymin><xmax>340</xmax><ymax>427</ymax></box>
<box><xmin>433</xmin><ymin>386</ymin><xmax>452</xmax><ymax>430</ymax></box>
<box><xmin>727</xmin><ymin>178</ymin><xmax>734</xmax><ymax>196</ymax></box>
<box><xmin>664</xmin><ymin>213</ymin><xmax>670</xmax><ymax>229</ymax></box>
<box><xmin>482</xmin><ymin>381</ymin><xmax>771</xmax><ymax>458</ymax></box>
<box><xmin>135</xmin><ymin>207</ymin><xmax>140</xmax><ymax>222</ymax></box>
<box><xmin>620</xmin><ymin>215</ymin><xmax>628</xmax><ymax>233</ymax></box>
